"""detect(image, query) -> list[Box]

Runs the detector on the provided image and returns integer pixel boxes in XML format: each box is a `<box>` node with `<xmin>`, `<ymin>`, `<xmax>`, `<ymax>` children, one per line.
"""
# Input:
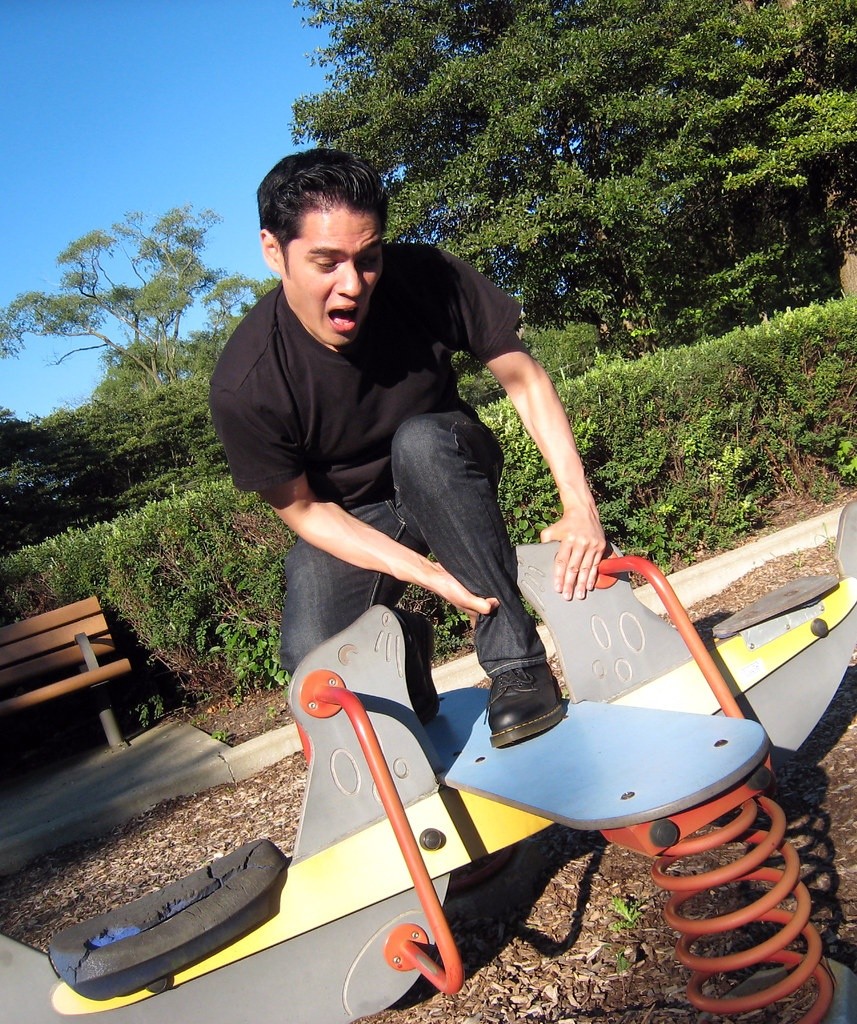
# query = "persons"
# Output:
<box><xmin>209</xmin><ymin>150</ymin><xmax>607</xmax><ymax>748</ymax></box>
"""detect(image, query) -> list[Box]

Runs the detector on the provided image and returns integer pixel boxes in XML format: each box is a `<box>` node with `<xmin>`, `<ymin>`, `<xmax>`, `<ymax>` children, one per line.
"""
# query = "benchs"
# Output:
<box><xmin>0</xmin><ymin>594</ymin><xmax>144</xmax><ymax>760</ymax></box>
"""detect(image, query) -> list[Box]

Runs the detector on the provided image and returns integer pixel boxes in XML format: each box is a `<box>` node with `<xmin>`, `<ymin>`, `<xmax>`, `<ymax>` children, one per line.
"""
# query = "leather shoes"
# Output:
<box><xmin>485</xmin><ymin>659</ymin><xmax>567</xmax><ymax>744</ymax></box>
